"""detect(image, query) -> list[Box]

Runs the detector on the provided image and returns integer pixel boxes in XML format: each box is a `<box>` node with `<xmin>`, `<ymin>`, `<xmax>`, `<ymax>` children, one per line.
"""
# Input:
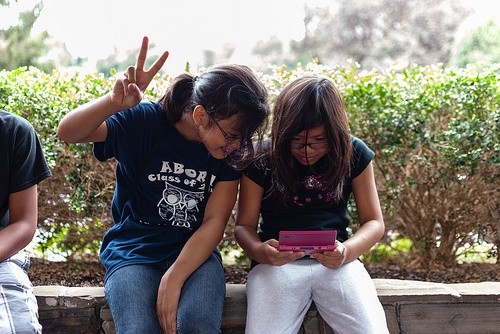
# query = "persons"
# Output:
<box><xmin>0</xmin><ymin>110</ymin><xmax>53</xmax><ymax>334</ymax></box>
<box><xmin>234</xmin><ymin>75</ymin><xmax>390</xmax><ymax>334</ymax></box>
<box><xmin>58</xmin><ymin>36</ymin><xmax>270</xmax><ymax>334</ymax></box>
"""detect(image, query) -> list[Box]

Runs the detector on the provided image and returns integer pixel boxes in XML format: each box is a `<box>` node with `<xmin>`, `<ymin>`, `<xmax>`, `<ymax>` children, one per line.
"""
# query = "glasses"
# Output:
<box><xmin>290</xmin><ymin>141</ymin><xmax>330</xmax><ymax>150</ymax></box>
<box><xmin>210</xmin><ymin>114</ymin><xmax>252</xmax><ymax>143</ymax></box>
